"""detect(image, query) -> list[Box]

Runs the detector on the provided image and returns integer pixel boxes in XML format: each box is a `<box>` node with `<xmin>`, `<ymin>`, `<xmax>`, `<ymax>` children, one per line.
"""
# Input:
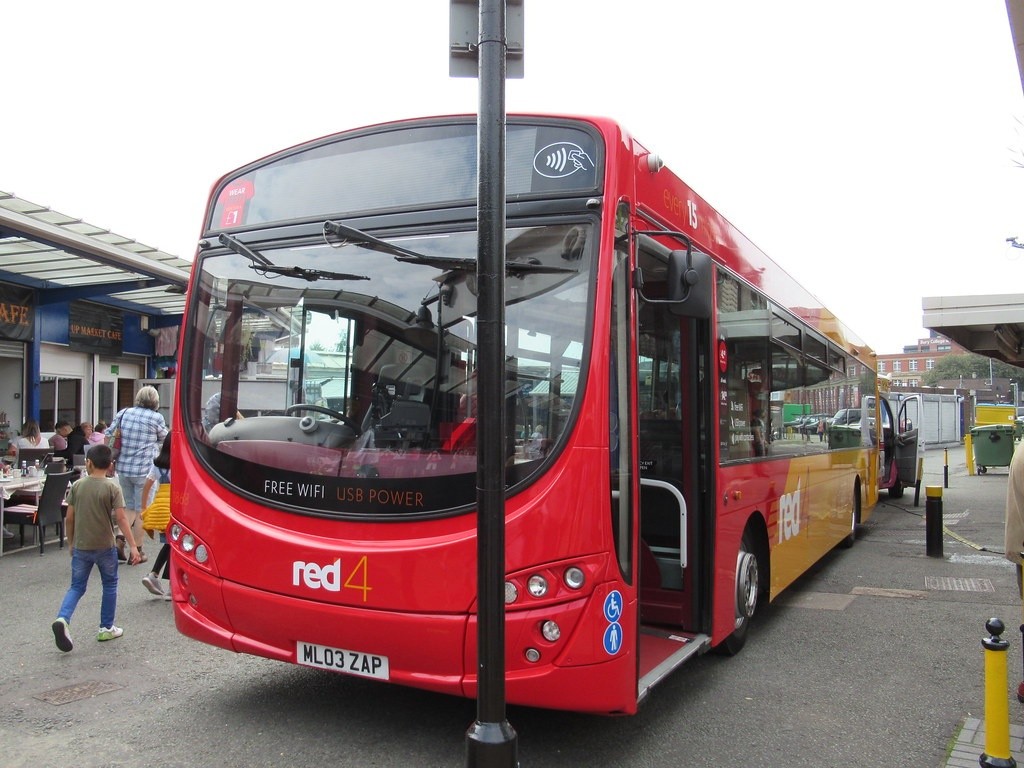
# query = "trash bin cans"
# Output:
<box><xmin>828</xmin><ymin>426</ymin><xmax>861</xmax><ymax>450</ymax></box>
<box><xmin>969</xmin><ymin>424</ymin><xmax>1016</xmax><ymax>476</ymax></box>
<box><xmin>1014</xmin><ymin>420</ymin><xmax>1023</xmax><ymax>441</ymax></box>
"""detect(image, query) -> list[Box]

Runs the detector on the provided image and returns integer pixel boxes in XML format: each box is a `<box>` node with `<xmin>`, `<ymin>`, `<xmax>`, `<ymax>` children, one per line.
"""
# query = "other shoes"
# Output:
<box><xmin>128</xmin><ymin>553</ymin><xmax>148</xmax><ymax>564</ymax></box>
<box><xmin>115</xmin><ymin>538</ymin><xmax>127</xmax><ymax>561</ymax></box>
<box><xmin>3</xmin><ymin>528</ymin><xmax>14</xmax><ymax>539</ymax></box>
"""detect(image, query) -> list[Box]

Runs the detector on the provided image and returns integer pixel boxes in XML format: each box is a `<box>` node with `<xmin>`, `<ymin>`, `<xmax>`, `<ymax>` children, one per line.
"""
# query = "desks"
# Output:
<box><xmin>0</xmin><ymin>475</ymin><xmax>46</xmax><ymax>556</ymax></box>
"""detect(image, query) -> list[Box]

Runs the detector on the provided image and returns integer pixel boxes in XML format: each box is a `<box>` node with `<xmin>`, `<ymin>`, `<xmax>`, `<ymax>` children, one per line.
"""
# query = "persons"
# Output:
<box><xmin>52</xmin><ymin>444</ymin><xmax>141</xmax><ymax>653</ymax></box>
<box><xmin>0</xmin><ymin>386</ymin><xmax>244</xmax><ymax>602</ymax></box>
<box><xmin>1004</xmin><ymin>437</ymin><xmax>1024</xmax><ymax>702</ymax></box>
<box><xmin>816</xmin><ymin>419</ymin><xmax>825</xmax><ymax>441</ymax></box>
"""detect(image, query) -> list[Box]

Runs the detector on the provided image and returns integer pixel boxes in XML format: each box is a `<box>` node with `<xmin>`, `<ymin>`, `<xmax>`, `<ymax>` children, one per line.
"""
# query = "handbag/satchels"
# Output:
<box><xmin>109</xmin><ymin>408</ymin><xmax>128</xmax><ymax>461</ymax></box>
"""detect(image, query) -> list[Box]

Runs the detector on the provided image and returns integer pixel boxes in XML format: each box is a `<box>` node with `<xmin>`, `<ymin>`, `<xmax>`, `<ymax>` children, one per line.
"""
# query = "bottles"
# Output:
<box><xmin>21</xmin><ymin>460</ymin><xmax>26</xmax><ymax>477</ymax></box>
<box><xmin>34</xmin><ymin>459</ymin><xmax>39</xmax><ymax>471</ymax></box>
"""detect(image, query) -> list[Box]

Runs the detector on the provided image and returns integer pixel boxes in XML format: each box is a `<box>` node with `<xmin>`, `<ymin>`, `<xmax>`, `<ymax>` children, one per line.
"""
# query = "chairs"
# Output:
<box><xmin>360</xmin><ymin>364</ymin><xmax>428</xmax><ymax>444</ymax></box>
<box><xmin>4</xmin><ymin>472</ymin><xmax>72</xmax><ymax>556</ymax></box>
<box><xmin>18</xmin><ymin>448</ymin><xmax>55</xmax><ymax>468</ymax></box>
<box><xmin>44</xmin><ymin>463</ymin><xmax>64</xmax><ymax>474</ymax></box>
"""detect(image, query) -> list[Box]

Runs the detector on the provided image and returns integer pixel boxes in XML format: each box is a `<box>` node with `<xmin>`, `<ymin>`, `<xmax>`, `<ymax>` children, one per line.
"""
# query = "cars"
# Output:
<box><xmin>779</xmin><ymin>407</ymin><xmax>876</xmax><ymax>439</ymax></box>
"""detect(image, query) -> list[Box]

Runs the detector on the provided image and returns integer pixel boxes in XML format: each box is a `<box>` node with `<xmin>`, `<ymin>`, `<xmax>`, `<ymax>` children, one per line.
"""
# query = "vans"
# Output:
<box><xmin>195</xmin><ymin>375</ymin><xmax>330</xmax><ymax>438</ymax></box>
<box><xmin>875</xmin><ymin>373</ymin><xmax>923</xmax><ymax>500</ymax></box>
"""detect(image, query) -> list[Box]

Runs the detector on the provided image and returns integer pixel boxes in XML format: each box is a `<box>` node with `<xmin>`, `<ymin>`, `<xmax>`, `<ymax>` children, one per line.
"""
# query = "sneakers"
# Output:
<box><xmin>52</xmin><ymin>618</ymin><xmax>73</xmax><ymax>651</ymax></box>
<box><xmin>142</xmin><ymin>573</ymin><xmax>164</xmax><ymax>595</ymax></box>
<box><xmin>165</xmin><ymin>592</ymin><xmax>172</xmax><ymax>600</ymax></box>
<box><xmin>98</xmin><ymin>625</ymin><xmax>123</xmax><ymax>640</ymax></box>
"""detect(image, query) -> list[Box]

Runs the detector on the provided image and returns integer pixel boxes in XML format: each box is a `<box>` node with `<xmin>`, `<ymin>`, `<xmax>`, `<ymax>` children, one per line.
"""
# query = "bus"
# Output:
<box><xmin>165</xmin><ymin>111</ymin><xmax>875</xmax><ymax>718</ymax></box>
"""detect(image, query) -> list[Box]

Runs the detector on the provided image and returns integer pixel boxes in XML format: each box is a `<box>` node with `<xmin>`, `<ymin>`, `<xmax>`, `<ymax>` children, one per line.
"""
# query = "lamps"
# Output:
<box><xmin>164</xmin><ymin>285</ymin><xmax>186</xmax><ymax>295</ymax></box>
